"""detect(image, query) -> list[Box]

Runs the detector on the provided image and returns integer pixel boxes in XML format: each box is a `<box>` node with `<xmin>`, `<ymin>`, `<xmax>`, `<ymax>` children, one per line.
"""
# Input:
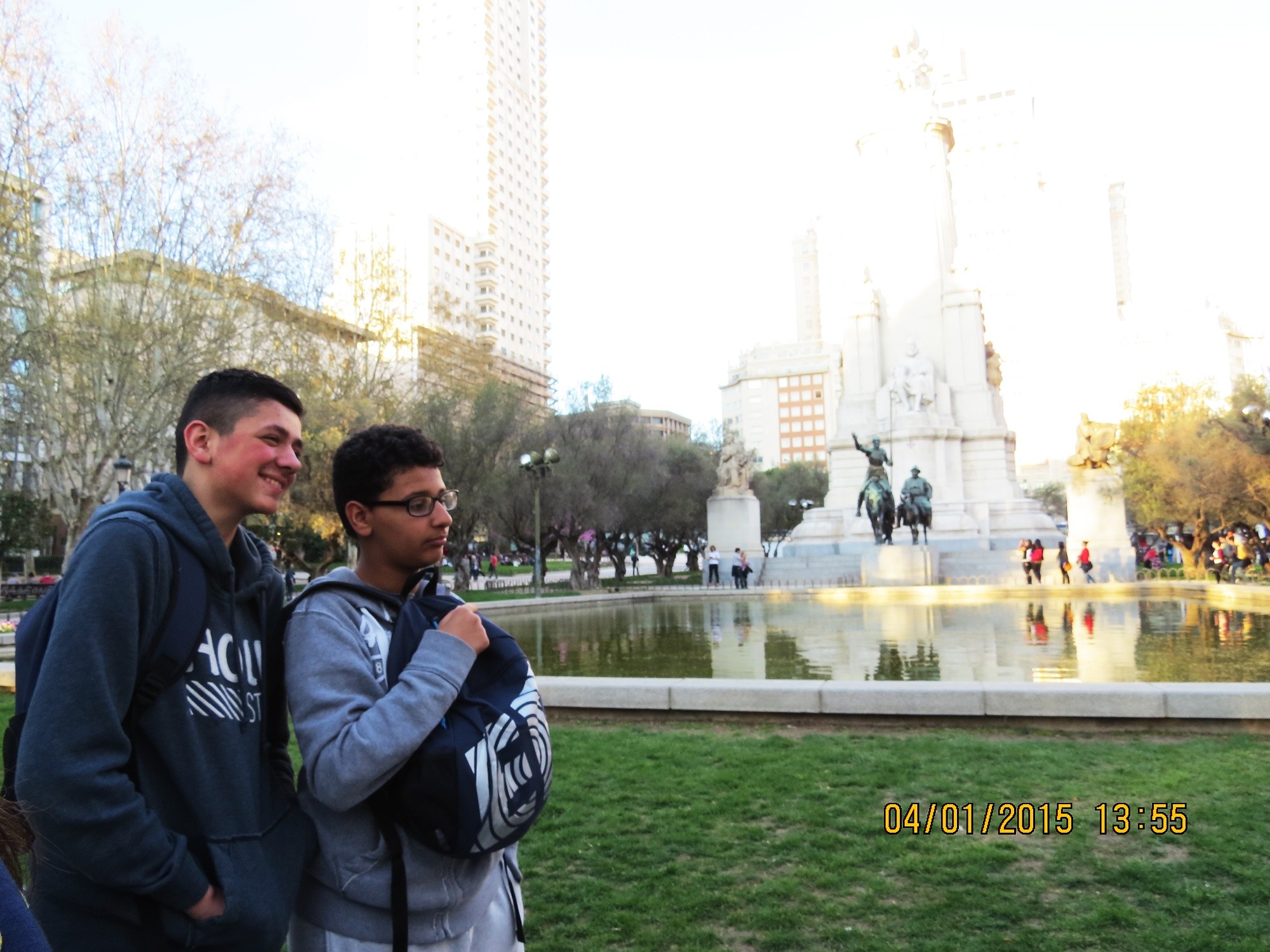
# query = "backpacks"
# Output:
<box><xmin>3</xmin><ymin>525</ymin><xmax>205</xmax><ymax>792</ymax></box>
<box><xmin>284</xmin><ymin>565</ymin><xmax>554</xmax><ymax>859</ymax></box>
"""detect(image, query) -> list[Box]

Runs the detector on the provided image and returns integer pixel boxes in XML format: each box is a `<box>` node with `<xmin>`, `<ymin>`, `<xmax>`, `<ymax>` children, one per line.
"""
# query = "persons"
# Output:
<box><xmin>434</xmin><ymin>551</ymin><xmax>498</xmax><ymax>581</ymax></box>
<box><xmin>285</xmin><ymin>563</ymin><xmax>297</xmax><ymax>604</ymax></box>
<box><xmin>1018</xmin><ymin>525</ymin><xmax>1269</xmax><ymax>586</ymax></box>
<box><xmin>7</xmin><ymin>367</ymin><xmax>318</xmax><ymax>952</ymax></box>
<box><xmin>731</xmin><ymin>547</ymin><xmax>753</xmax><ymax>590</ymax></box>
<box><xmin>850</xmin><ymin>433</ymin><xmax>895</xmax><ymax>517</ymax></box>
<box><xmin>278</xmin><ymin>423</ymin><xmax>525</xmax><ymax>951</ymax></box>
<box><xmin>891</xmin><ymin>466</ymin><xmax>932</xmax><ymax>530</ymax></box>
<box><xmin>707</xmin><ymin>545</ymin><xmax>721</xmax><ymax>587</ymax></box>
<box><xmin>5</xmin><ymin>571</ymin><xmax>65</xmax><ymax>602</ymax></box>
<box><xmin>631</xmin><ymin>552</ymin><xmax>641</xmax><ymax>577</ymax></box>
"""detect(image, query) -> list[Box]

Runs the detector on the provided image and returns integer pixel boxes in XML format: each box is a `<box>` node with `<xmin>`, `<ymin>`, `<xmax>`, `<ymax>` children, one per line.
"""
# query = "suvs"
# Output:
<box><xmin>1135</xmin><ymin>532</ymin><xmax>1160</xmax><ymax>563</ymax></box>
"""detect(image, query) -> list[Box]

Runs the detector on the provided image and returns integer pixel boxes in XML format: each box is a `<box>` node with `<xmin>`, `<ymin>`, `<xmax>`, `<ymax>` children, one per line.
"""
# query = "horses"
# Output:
<box><xmin>901</xmin><ymin>493</ymin><xmax>931</xmax><ymax>546</ymax></box>
<box><xmin>866</xmin><ymin>481</ymin><xmax>895</xmax><ymax>545</ymax></box>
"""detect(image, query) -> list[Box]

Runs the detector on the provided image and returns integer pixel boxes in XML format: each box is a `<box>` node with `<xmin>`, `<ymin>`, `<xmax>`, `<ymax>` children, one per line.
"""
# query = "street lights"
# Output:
<box><xmin>111</xmin><ymin>455</ymin><xmax>133</xmax><ymax>495</ymax></box>
<box><xmin>517</xmin><ymin>447</ymin><xmax>561</xmax><ymax>596</ymax></box>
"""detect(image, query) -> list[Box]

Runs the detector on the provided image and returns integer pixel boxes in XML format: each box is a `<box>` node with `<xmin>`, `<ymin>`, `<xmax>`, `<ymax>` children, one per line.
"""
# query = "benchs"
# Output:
<box><xmin>0</xmin><ymin>584</ymin><xmax>53</xmax><ymax>602</ymax></box>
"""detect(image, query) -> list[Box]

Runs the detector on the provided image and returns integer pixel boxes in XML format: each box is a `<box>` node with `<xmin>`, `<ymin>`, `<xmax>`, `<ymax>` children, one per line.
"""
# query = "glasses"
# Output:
<box><xmin>366</xmin><ymin>487</ymin><xmax>458</xmax><ymax>516</ymax></box>
